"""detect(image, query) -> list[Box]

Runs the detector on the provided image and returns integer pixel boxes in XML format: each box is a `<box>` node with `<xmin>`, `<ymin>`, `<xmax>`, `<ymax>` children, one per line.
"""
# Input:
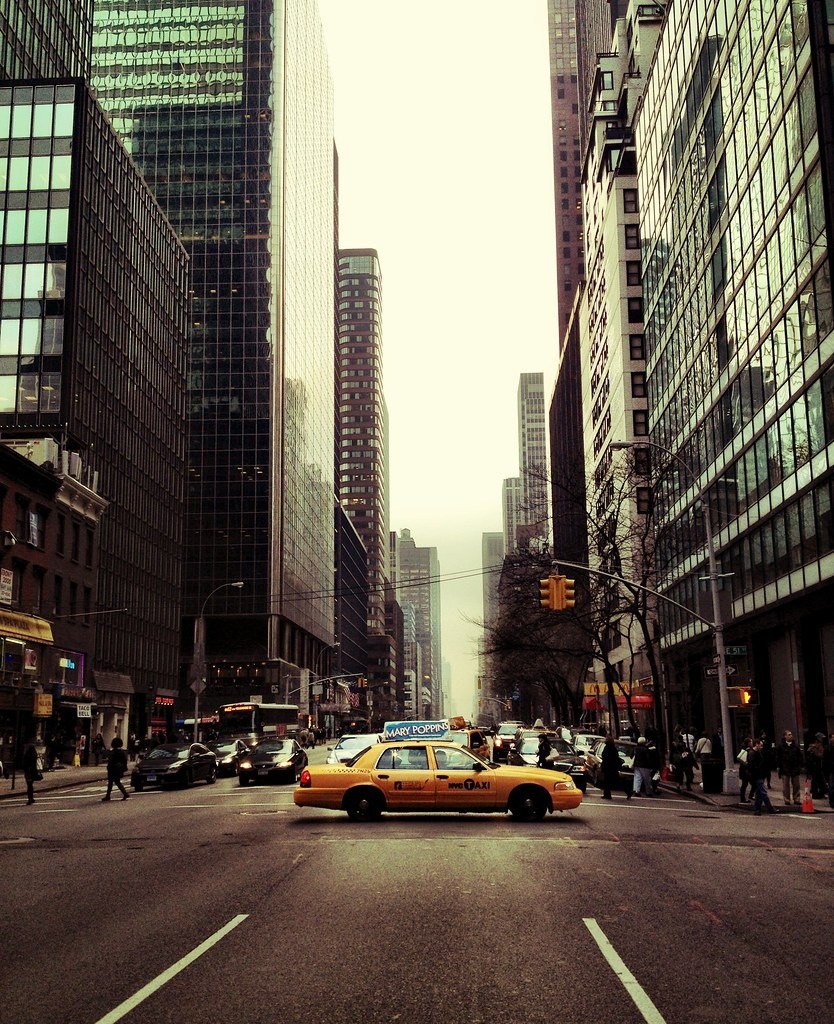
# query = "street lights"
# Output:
<box><xmin>316</xmin><ymin>642</ymin><xmax>340</xmax><ymax>723</ymax></box>
<box><xmin>609</xmin><ymin>440</ymin><xmax>741</xmax><ymax>796</ymax></box>
<box><xmin>193</xmin><ymin>580</ymin><xmax>244</xmax><ymax>741</ymax></box>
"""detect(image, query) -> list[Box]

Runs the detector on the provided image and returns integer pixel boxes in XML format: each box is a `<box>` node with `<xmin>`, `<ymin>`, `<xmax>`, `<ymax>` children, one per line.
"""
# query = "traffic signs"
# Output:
<box><xmin>704</xmin><ymin>646</ymin><xmax>749</xmax><ymax>677</ymax></box>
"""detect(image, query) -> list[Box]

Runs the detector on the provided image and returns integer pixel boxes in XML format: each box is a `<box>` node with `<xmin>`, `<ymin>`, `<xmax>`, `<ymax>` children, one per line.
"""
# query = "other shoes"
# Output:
<box><xmin>102</xmin><ymin>796</ymin><xmax>110</xmax><ymax>801</ymax></box>
<box><xmin>754</xmin><ymin>810</ymin><xmax>761</xmax><ymax>815</ymax></box>
<box><xmin>635</xmin><ymin>792</ymin><xmax>641</xmax><ymax>797</ymax></box>
<box><xmin>830</xmin><ymin>802</ymin><xmax>834</xmax><ymax>808</ymax></box>
<box><xmin>784</xmin><ymin>797</ymin><xmax>790</xmax><ymax>804</ymax></box>
<box><xmin>601</xmin><ymin>796</ymin><xmax>612</xmax><ymax>799</ymax></box>
<box><xmin>27</xmin><ymin>799</ymin><xmax>35</xmax><ymax>805</ymax></box>
<box><xmin>748</xmin><ymin>796</ymin><xmax>755</xmax><ymax>800</ymax></box>
<box><xmin>769</xmin><ymin>809</ymin><xmax>780</xmax><ymax>813</ymax></box>
<box><xmin>812</xmin><ymin>792</ymin><xmax>827</xmax><ymax>799</ymax></box>
<box><xmin>741</xmin><ymin>800</ymin><xmax>751</xmax><ymax>803</ymax></box>
<box><xmin>121</xmin><ymin>792</ymin><xmax>129</xmax><ymax>800</ymax></box>
<box><xmin>627</xmin><ymin>791</ymin><xmax>634</xmax><ymax>800</ymax></box>
<box><xmin>646</xmin><ymin>791</ymin><xmax>654</xmax><ymax>796</ymax></box>
<box><xmin>794</xmin><ymin>800</ymin><xmax>801</xmax><ymax>805</ymax></box>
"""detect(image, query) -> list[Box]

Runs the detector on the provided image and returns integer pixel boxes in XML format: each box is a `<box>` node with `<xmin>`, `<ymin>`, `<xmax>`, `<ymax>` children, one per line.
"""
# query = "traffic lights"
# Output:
<box><xmin>743</xmin><ymin>688</ymin><xmax>760</xmax><ymax>704</ymax></box>
<box><xmin>565</xmin><ymin>579</ymin><xmax>577</xmax><ymax>607</ymax></box>
<box><xmin>364</xmin><ymin>679</ymin><xmax>367</xmax><ymax>687</ymax></box>
<box><xmin>538</xmin><ymin>578</ymin><xmax>551</xmax><ymax>608</ymax></box>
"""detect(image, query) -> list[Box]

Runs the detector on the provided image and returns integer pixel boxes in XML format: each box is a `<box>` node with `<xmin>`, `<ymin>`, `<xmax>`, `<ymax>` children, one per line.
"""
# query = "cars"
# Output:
<box><xmin>204</xmin><ymin>739</ymin><xmax>252</xmax><ymax>776</ymax></box>
<box><xmin>238</xmin><ymin>739</ymin><xmax>309</xmax><ymax>785</ymax></box>
<box><xmin>131</xmin><ymin>742</ymin><xmax>217</xmax><ymax>789</ymax></box>
<box><xmin>293</xmin><ymin>716</ymin><xmax>660</xmax><ymax>820</ymax></box>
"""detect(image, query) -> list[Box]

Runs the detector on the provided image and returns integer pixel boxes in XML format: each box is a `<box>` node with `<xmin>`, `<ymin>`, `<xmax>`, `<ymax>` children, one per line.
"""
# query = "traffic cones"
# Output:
<box><xmin>801</xmin><ymin>779</ymin><xmax>816</xmax><ymax>812</ymax></box>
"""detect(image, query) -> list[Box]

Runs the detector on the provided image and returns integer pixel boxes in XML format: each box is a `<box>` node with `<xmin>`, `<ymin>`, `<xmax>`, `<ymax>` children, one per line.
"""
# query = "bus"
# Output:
<box><xmin>218</xmin><ymin>702</ymin><xmax>300</xmax><ymax>746</ymax></box>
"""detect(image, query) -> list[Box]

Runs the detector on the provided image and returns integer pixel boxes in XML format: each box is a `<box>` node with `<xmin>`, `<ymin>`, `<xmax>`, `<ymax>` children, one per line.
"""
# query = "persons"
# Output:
<box><xmin>436</xmin><ymin>751</ymin><xmax>451</xmax><ymax>770</ymax></box>
<box><xmin>534</xmin><ymin>720</ymin><xmax>834</xmax><ymax>815</ymax></box>
<box><xmin>301</xmin><ymin>723</ymin><xmax>385</xmax><ymax>749</ymax></box>
<box><xmin>408</xmin><ymin>749</ymin><xmax>423</xmax><ymax>765</ymax></box>
<box><xmin>21</xmin><ymin>728</ymin><xmax>218</xmax><ymax>806</ymax></box>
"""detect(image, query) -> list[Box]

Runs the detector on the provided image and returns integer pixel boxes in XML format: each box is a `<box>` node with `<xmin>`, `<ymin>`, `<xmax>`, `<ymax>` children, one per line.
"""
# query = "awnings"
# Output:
<box><xmin>583</xmin><ymin>692</ymin><xmax>656</xmax><ymax>710</ymax></box>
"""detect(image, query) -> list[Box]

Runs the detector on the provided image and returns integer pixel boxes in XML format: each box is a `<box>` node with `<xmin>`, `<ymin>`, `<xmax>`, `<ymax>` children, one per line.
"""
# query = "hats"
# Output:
<box><xmin>815</xmin><ymin>733</ymin><xmax>825</xmax><ymax>738</ymax></box>
<box><xmin>536</xmin><ymin>734</ymin><xmax>547</xmax><ymax>738</ymax></box>
<box><xmin>638</xmin><ymin>737</ymin><xmax>646</xmax><ymax>744</ymax></box>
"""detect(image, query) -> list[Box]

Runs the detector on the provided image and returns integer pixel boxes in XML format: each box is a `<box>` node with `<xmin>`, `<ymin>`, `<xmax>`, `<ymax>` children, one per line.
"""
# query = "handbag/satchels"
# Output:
<box><xmin>736</xmin><ymin>747</ymin><xmax>751</xmax><ymax>765</ymax></box>
<box><xmin>616</xmin><ymin>758</ymin><xmax>625</xmax><ymax>768</ymax></box>
<box><xmin>34</xmin><ymin>771</ymin><xmax>43</xmax><ymax>780</ymax></box>
